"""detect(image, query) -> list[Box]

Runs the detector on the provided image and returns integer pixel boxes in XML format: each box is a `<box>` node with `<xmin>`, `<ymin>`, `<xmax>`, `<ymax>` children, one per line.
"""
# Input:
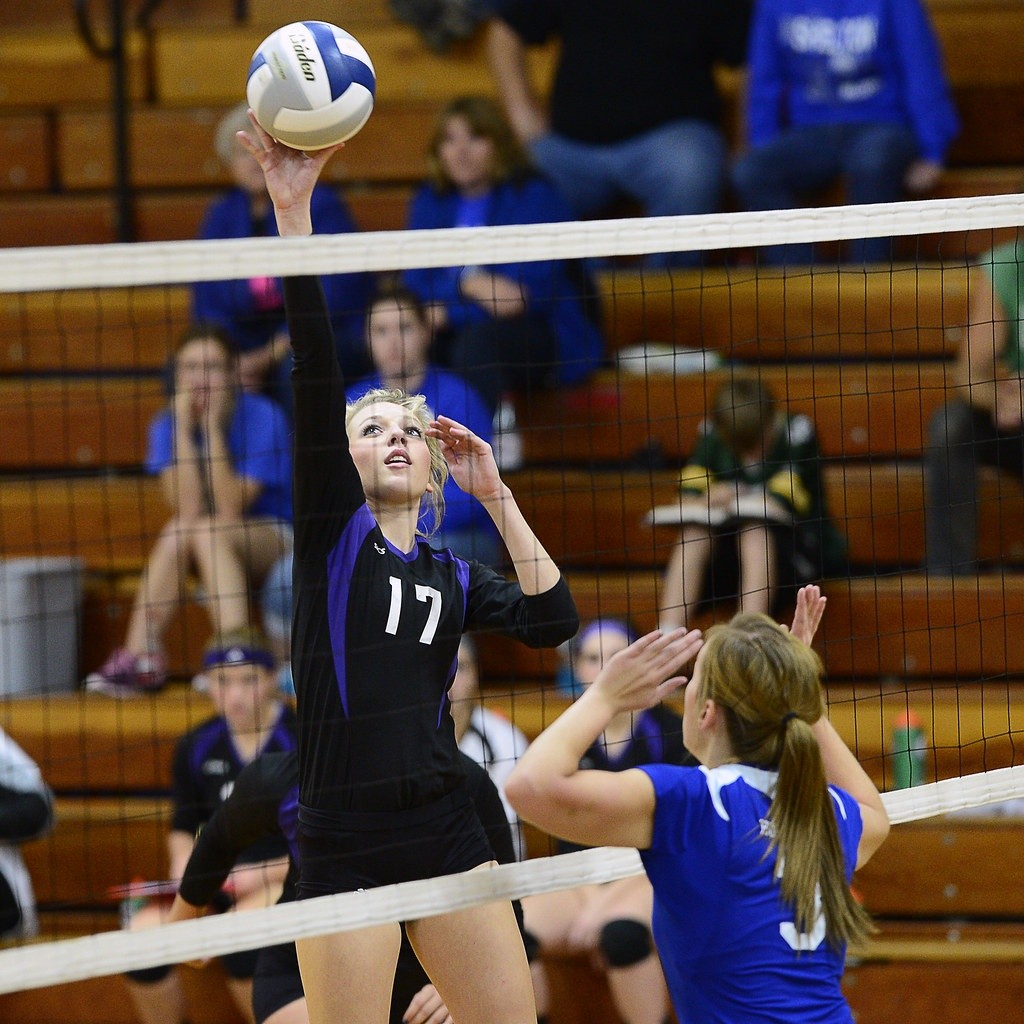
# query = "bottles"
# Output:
<box><xmin>891</xmin><ymin>707</ymin><xmax>927</xmax><ymax>792</ymax></box>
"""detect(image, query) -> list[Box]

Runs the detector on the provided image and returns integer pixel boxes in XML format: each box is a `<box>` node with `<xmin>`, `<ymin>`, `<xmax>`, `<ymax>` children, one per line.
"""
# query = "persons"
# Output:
<box><xmin>228</xmin><ymin>100</ymin><xmax>584</xmax><ymax>1024</ymax></box>
<box><xmin>656</xmin><ymin>373</ymin><xmax>850</xmax><ymax>647</ymax></box>
<box><xmin>79</xmin><ymin>321</ymin><xmax>301</xmax><ymax>701</ymax></box>
<box><xmin>0</xmin><ymin>612</ymin><xmax>692</xmax><ymax>1023</ymax></box>
<box><xmin>502</xmin><ymin>579</ymin><xmax>892</xmax><ymax>1024</ymax></box>
<box><xmin>344</xmin><ymin>289</ymin><xmax>519</xmax><ymax>595</ymax></box>
<box><xmin>193</xmin><ymin>101</ymin><xmax>377</xmax><ymax>372</ymax></box>
<box><xmin>727</xmin><ymin>1</ymin><xmax>956</xmax><ymax>266</ymax></box>
<box><xmin>470</xmin><ymin>2</ymin><xmax>741</xmax><ymax>270</ymax></box>
<box><xmin>391</xmin><ymin>93</ymin><xmax>614</xmax><ymax>473</ymax></box>
<box><xmin>911</xmin><ymin>237</ymin><xmax>1024</xmax><ymax>579</ymax></box>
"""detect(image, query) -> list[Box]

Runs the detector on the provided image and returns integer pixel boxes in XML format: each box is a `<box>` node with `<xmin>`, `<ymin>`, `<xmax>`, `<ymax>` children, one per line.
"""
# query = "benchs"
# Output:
<box><xmin>0</xmin><ymin>0</ymin><xmax>1024</xmax><ymax>675</ymax></box>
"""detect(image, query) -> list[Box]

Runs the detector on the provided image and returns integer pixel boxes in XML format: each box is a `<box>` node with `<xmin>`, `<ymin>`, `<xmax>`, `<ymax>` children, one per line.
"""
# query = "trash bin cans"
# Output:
<box><xmin>0</xmin><ymin>554</ymin><xmax>91</xmax><ymax>702</ymax></box>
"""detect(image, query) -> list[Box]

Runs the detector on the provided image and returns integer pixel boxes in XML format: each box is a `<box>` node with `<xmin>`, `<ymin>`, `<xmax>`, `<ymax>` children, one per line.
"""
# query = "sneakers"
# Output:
<box><xmin>85</xmin><ymin>649</ymin><xmax>170</xmax><ymax>698</ymax></box>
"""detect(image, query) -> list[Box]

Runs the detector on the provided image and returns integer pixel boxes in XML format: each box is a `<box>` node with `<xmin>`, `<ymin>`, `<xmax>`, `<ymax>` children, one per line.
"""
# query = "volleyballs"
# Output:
<box><xmin>245</xmin><ymin>20</ymin><xmax>376</xmax><ymax>151</ymax></box>
